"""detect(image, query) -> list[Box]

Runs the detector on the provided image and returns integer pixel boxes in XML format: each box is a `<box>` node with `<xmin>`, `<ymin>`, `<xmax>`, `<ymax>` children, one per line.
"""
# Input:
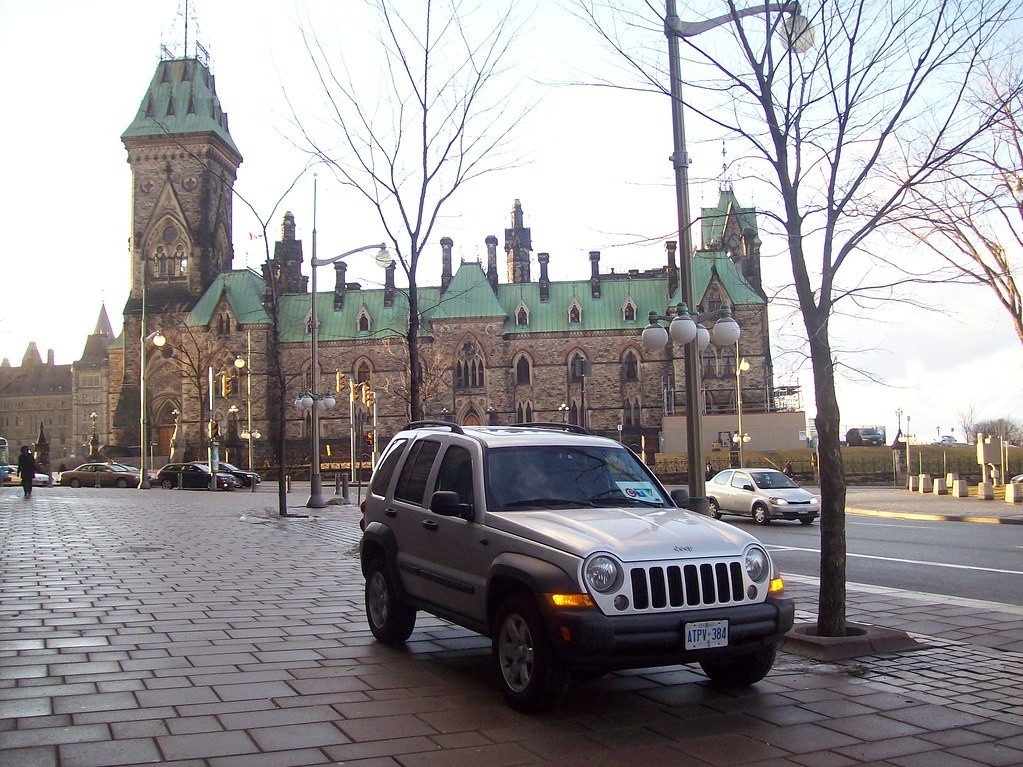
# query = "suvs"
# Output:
<box><xmin>359</xmin><ymin>421</ymin><xmax>795</xmax><ymax>719</ymax></box>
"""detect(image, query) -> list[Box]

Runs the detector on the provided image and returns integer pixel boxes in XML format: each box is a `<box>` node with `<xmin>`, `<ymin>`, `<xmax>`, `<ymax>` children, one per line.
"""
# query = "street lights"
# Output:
<box><xmin>641</xmin><ymin>0</ymin><xmax>816</xmax><ymax>513</ymax></box>
<box><xmin>292</xmin><ymin>178</ymin><xmax>394</xmax><ymax>509</ymax></box>
<box><xmin>893</xmin><ymin>408</ymin><xmax>906</xmax><ymax>450</ymax></box>
<box><xmin>936</xmin><ymin>425</ymin><xmax>940</xmax><ymax>436</ymax></box>
<box><xmin>89</xmin><ymin>412</ymin><xmax>100</xmax><ymax>459</ymax></box>
<box><xmin>234</xmin><ymin>330</ymin><xmax>262</xmax><ymax>471</ymax></box>
<box><xmin>733</xmin><ymin>340</ymin><xmax>753</xmax><ymax>468</ymax></box>
<box><xmin>138</xmin><ymin>289</ymin><xmax>166</xmax><ymax>489</ymax></box>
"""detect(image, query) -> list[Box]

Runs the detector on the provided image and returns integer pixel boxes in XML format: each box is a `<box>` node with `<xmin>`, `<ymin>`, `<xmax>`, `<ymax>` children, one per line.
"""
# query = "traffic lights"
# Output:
<box><xmin>366</xmin><ymin>430</ymin><xmax>373</xmax><ymax>441</ymax></box>
<box><xmin>336</xmin><ymin>371</ymin><xmax>345</xmax><ymax>393</ymax></box>
<box><xmin>367</xmin><ymin>392</ymin><xmax>376</xmax><ymax>408</ymax></box>
<box><xmin>362</xmin><ymin>384</ymin><xmax>370</xmax><ymax>403</ymax></box>
<box><xmin>208</xmin><ymin>421</ymin><xmax>217</xmax><ymax>439</ymax></box>
<box><xmin>221</xmin><ymin>374</ymin><xmax>231</xmax><ymax>398</ymax></box>
<box><xmin>350</xmin><ymin>379</ymin><xmax>359</xmax><ymax>402</ymax></box>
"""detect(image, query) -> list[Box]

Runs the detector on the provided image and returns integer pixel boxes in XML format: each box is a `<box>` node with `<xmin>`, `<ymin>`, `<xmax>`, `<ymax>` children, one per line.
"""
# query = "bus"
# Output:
<box><xmin>0</xmin><ymin>437</ymin><xmax>8</xmax><ymax>466</ymax></box>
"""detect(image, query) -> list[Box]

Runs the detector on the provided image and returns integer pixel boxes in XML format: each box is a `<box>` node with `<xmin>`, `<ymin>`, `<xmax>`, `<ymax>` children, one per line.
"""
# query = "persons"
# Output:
<box><xmin>17</xmin><ymin>446</ymin><xmax>44</xmax><ymax>499</ymax></box>
<box><xmin>786</xmin><ymin>461</ymin><xmax>794</xmax><ymax>481</ymax></box>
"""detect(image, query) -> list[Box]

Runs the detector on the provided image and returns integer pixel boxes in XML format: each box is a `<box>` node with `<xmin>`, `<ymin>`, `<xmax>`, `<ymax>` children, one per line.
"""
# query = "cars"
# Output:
<box><xmin>705</xmin><ymin>468</ymin><xmax>821</xmax><ymax>526</ymax></box>
<box><xmin>155</xmin><ymin>463</ymin><xmax>237</xmax><ymax>491</ymax></box>
<box><xmin>0</xmin><ymin>464</ymin><xmax>50</xmax><ymax>486</ymax></box>
<box><xmin>184</xmin><ymin>461</ymin><xmax>261</xmax><ymax>488</ymax></box>
<box><xmin>59</xmin><ymin>463</ymin><xmax>140</xmax><ymax>488</ymax></box>
<box><xmin>845</xmin><ymin>427</ymin><xmax>883</xmax><ymax>447</ymax></box>
<box><xmin>1010</xmin><ymin>474</ymin><xmax>1023</xmax><ymax>484</ymax></box>
